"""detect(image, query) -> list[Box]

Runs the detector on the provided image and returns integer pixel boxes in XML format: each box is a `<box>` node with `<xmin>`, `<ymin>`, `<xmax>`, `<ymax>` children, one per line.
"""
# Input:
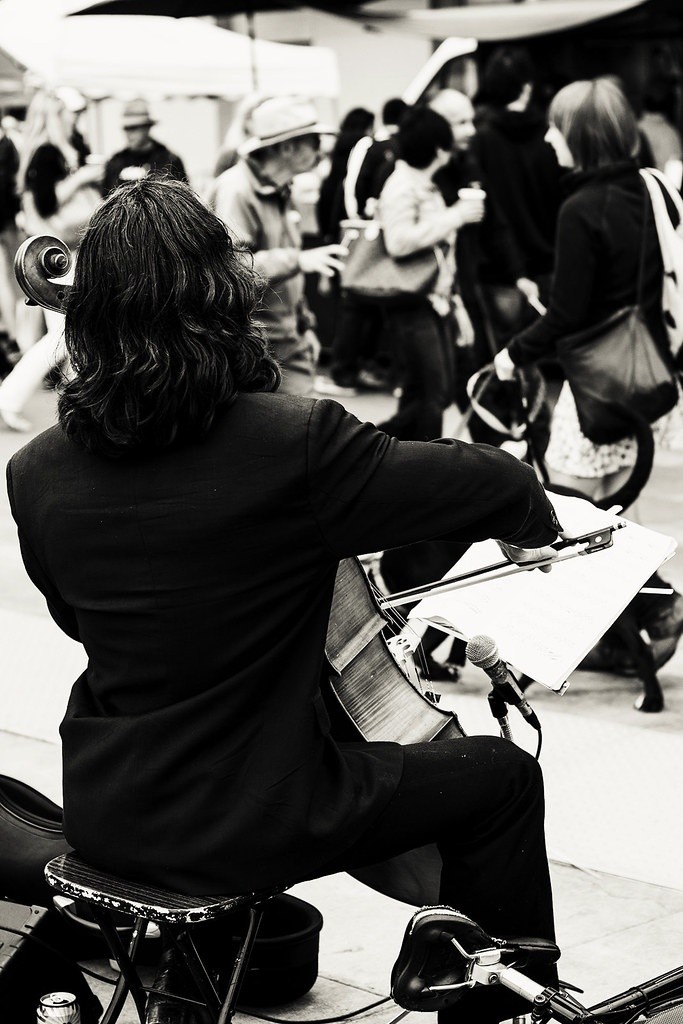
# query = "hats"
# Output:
<box><xmin>122</xmin><ymin>99</ymin><xmax>155</xmax><ymax>129</ymax></box>
<box><xmin>55</xmin><ymin>86</ymin><xmax>86</xmax><ymax>112</ymax></box>
<box><xmin>236</xmin><ymin>98</ymin><xmax>342</xmax><ymax>155</ymax></box>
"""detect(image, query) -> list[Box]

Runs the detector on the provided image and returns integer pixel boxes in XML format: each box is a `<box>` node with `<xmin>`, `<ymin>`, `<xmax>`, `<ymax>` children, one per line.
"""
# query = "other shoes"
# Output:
<box><xmin>313</xmin><ymin>375</ymin><xmax>356</xmax><ymax>397</ymax></box>
<box><xmin>3</xmin><ymin>410</ymin><xmax>31</xmax><ymax>431</ymax></box>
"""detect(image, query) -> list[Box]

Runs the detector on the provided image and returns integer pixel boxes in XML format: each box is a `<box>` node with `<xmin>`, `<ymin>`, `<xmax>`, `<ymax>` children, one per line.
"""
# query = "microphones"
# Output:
<box><xmin>465</xmin><ymin>635</ymin><xmax>541</xmax><ymax>732</ymax></box>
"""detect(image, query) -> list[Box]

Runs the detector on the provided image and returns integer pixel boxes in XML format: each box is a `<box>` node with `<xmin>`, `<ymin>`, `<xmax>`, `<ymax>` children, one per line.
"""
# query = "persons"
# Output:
<box><xmin>2</xmin><ymin>175</ymin><xmax>565</xmax><ymax>1022</ymax></box>
<box><xmin>194</xmin><ymin>94</ymin><xmax>351</xmax><ymax>397</ymax></box>
<box><xmin>366</xmin><ymin>106</ymin><xmax>488</xmax><ymax>442</ymax></box>
<box><xmin>425</xmin><ymin>56</ymin><xmax>479</xmax><ymax>103</ymax></box>
<box><xmin>312</xmin><ymin>108</ymin><xmax>378</xmax><ymax>384</ymax></box>
<box><xmin>426</xmin><ymin>87</ymin><xmax>503</xmax><ymax>446</ymax></box>
<box><xmin>212</xmin><ymin>93</ymin><xmax>273</xmax><ymax>176</ymax></box>
<box><xmin>341</xmin><ymin>95</ymin><xmax>410</xmax><ymax>393</ymax></box>
<box><xmin>100</xmin><ymin>99</ymin><xmax>189</xmax><ymax>206</ymax></box>
<box><xmin>1</xmin><ymin>88</ymin><xmax>106</xmax><ymax>435</ymax></box>
<box><xmin>492</xmin><ymin>73</ymin><xmax>683</xmax><ymax>502</ymax></box>
<box><xmin>481</xmin><ymin>46</ymin><xmax>556</xmax><ymax>312</ymax></box>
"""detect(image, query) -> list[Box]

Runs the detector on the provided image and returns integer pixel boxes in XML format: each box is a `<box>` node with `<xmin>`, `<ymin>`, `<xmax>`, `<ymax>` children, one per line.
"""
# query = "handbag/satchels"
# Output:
<box><xmin>554</xmin><ymin>306</ymin><xmax>680</xmax><ymax>446</ymax></box>
<box><xmin>340</xmin><ymin>219</ymin><xmax>450</xmax><ymax>297</ymax></box>
<box><xmin>640</xmin><ymin>167</ymin><xmax>683</xmax><ymax>353</ymax></box>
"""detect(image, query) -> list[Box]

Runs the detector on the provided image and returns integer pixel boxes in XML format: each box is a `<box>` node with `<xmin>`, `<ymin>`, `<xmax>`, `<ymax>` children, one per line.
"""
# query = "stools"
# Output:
<box><xmin>43</xmin><ymin>849</ymin><xmax>294</xmax><ymax>1024</ymax></box>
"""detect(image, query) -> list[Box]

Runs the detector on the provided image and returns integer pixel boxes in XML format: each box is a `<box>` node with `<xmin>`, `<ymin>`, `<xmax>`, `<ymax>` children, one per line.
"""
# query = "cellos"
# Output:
<box><xmin>15</xmin><ymin>233</ymin><xmax>476</xmax><ymax>910</ymax></box>
